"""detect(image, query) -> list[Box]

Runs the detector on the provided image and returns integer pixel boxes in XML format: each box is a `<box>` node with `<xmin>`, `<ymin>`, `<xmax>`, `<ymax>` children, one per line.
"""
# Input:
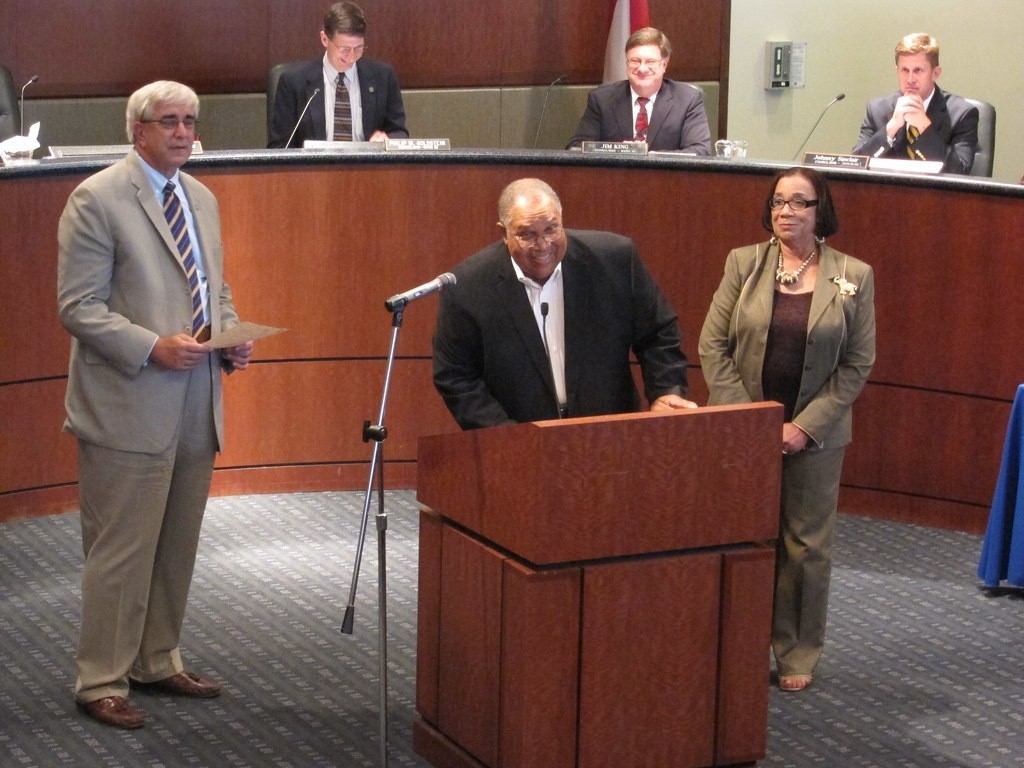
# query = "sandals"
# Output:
<box><xmin>779</xmin><ymin>670</ymin><xmax>812</xmax><ymax>692</ymax></box>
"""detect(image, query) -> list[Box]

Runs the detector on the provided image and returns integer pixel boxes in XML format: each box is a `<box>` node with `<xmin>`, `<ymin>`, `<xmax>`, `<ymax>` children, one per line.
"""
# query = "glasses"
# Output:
<box><xmin>328</xmin><ymin>35</ymin><xmax>368</xmax><ymax>56</ymax></box>
<box><xmin>135</xmin><ymin>116</ymin><xmax>199</xmax><ymax>130</ymax></box>
<box><xmin>767</xmin><ymin>194</ymin><xmax>819</xmax><ymax>212</ymax></box>
<box><xmin>504</xmin><ymin>221</ymin><xmax>563</xmax><ymax>250</ymax></box>
<box><xmin>625</xmin><ymin>56</ymin><xmax>664</xmax><ymax>69</ymax></box>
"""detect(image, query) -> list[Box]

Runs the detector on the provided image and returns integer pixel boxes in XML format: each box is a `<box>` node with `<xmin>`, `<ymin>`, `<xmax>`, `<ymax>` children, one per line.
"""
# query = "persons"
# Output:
<box><xmin>854</xmin><ymin>32</ymin><xmax>980</xmax><ymax>175</ymax></box>
<box><xmin>433</xmin><ymin>177</ymin><xmax>698</xmax><ymax>431</ymax></box>
<box><xmin>699</xmin><ymin>168</ymin><xmax>876</xmax><ymax>690</ymax></box>
<box><xmin>57</xmin><ymin>81</ymin><xmax>254</xmax><ymax>728</ymax></box>
<box><xmin>566</xmin><ymin>28</ymin><xmax>711</xmax><ymax>155</ymax></box>
<box><xmin>266</xmin><ymin>0</ymin><xmax>409</xmax><ymax>148</ymax></box>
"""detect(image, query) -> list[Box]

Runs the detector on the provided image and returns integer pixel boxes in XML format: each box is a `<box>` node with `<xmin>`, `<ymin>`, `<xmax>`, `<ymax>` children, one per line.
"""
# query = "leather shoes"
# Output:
<box><xmin>129</xmin><ymin>668</ymin><xmax>220</xmax><ymax>696</ymax></box>
<box><xmin>80</xmin><ymin>695</ymin><xmax>145</xmax><ymax>729</ymax></box>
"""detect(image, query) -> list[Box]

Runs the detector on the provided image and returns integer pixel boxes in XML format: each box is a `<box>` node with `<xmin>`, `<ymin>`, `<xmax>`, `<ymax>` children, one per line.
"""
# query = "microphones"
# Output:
<box><xmin>285</xmin><ymin>89</ymin><xmax>320</xmax><ymax>149</ymax></box>
<box><xmin>385</xmin><ymin>272</ymin><xmax>457</xmax><ymax>313</ymax></box>
<box><xmin>540</xmin><ymin>303</ymin><xmax>562</xmax><ymax>419</ymax></box>
<box><xmin>533</xmin><ymin>75</ymin><xmax>569</xmax><ymax>149</ymax></box>
<box><xmin>20</xmin><ymin>75</ymin><xmax>40</xmax><ymax>136</ymax></box>
<box><xmin>792</xmin><ymin>93</ymin><xmax>845</xmax><ymax>161</ymax></box>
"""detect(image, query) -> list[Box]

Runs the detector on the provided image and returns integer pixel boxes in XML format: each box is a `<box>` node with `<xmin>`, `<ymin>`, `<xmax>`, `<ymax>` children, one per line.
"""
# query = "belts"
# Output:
<box><xmin>196</xmin><ymin>325</ymin><xmax>212</xmax><ymax>342</ymax></box>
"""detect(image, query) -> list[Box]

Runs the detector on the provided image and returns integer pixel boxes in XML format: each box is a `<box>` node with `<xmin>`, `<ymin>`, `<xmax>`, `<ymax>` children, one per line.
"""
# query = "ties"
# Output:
<box><xmin>635</xmin><ymin>95</ymin><xmax>651</xmax><ymax>142</ymax></box>
<box><xmin>907</xmin><ymin>124</ymin><xmax>921</xmax><ymax>158</ymax></box>
<box><xmin>163</xmin><ymin>181</ymin><xmax>205</xmax><ymax>342</ymax></box>
<box><xmin>334</xmin><ymin>70</ymin><xmax>352</xmax><ymax>143</ymax></box>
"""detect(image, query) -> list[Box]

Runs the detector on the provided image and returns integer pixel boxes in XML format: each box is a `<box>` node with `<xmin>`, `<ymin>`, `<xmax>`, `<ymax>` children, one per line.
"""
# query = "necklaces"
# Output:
<box><xmin>776</xmin><ymin>247</ymin><xmax>816</xmax><ymax>284</ymax></box>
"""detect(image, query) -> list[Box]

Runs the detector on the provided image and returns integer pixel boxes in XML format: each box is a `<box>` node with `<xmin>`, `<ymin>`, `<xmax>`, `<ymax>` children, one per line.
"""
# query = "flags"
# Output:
<box><xmin>603</xmin><ymin>0</ymin><xmax>648</xmax><ymax>85</ymax></box>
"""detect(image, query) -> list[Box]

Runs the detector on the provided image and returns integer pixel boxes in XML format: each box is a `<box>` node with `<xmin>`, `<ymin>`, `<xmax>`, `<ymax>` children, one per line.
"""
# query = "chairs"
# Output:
<box><xmin>0</xmin><ymin>65</ymin><xmax>22</xmax><ymax>164</ymax></box>
<box><xmin>963</xmin><ymin>96</ymin><xmax>996</xmax><ymax>178</ymax></box>
<box><xmin>266</xmin><ymin>60</ymin><xmax>317</xmax><ymax>149</ymax></box>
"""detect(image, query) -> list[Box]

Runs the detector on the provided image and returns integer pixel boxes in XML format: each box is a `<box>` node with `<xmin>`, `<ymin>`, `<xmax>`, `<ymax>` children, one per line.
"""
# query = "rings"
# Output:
<box><xmin>783</xmin><ymin>451</ymin><xmax>788</xmax><ymax>454</ymax></box>
